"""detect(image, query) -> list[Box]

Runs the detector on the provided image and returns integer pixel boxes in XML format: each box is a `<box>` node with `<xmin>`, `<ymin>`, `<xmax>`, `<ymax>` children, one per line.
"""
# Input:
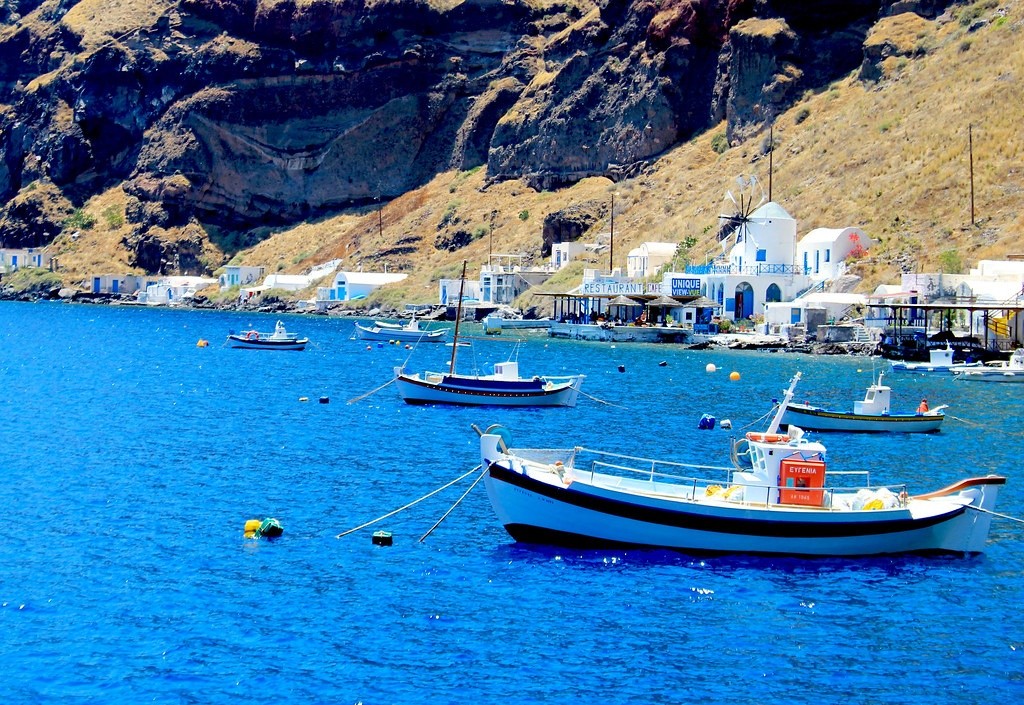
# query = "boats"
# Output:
<box><xmin>352</xmin><ymin>305</ymin><xmax>451</xmax><ymax>342</ymax></box>
<box><xmin>770</xmin><ymin>370</ymin><xmax>949</xmax><ymax>433</ymax></box>
<box><xmin>227</xmin><ymin>319</ymin><xmax>309</xmax><ymax>350</ymax></box>
<box><xmin>470</xmin><ymin>420</ymin><xmax>1008</xmax><ymax>559</ymax></box>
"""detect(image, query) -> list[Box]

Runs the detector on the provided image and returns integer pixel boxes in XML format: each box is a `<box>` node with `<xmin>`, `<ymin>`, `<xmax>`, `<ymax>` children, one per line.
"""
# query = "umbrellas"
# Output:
<box><xmin>605</xmin><ymin>295</ymin><xmax>642</xmax><ymax>318</ymax></box>
<box><xmin>683</xmin><ymin>295</ymin><xmax>724</xmax><ymax>308</ymax></box>
<box><xmin>645</xmin><ymin>295</ymin><xmax>684</xmax><ymax>316</ymax></box>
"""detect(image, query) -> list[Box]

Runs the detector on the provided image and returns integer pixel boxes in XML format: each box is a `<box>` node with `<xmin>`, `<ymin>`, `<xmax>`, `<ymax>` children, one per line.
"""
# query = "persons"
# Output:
<box><xmin>635</xmin><ymin>312</ymin><xmax>647</xmax><ymax>325</ymax></box>
<box><xmin>605</xmin><ymin>312</ymin><xmax>622</xmax><ymax>326</ymax></box>
<box><xmin>917</xmin><ymin>398</ymin><xmax>929</xmax><ymax>413</ymax></box>
<box><xmin>591</xmin><ymin>308</ymin><xmax>594</xmax><ymax>315</ymax></box>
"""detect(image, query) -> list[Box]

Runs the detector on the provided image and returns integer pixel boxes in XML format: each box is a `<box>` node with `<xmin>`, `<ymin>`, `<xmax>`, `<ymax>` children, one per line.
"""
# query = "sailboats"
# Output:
<box><xmin>392</xmin><ymin>258</ymin><xmax>587</xmax><ymax>407</ymax></box>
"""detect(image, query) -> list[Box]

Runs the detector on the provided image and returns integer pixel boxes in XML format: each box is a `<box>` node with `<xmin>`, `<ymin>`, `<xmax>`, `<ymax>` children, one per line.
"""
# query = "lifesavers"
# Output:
<box><xmin>745</xmin><ymin>431</ymin><xmax>790</xmax><ymax>443</ymax></box>
<box><xmin>246</xmin><ymin>330</ymin><xmax>259</xmax><ymax>340</ymax></box>
